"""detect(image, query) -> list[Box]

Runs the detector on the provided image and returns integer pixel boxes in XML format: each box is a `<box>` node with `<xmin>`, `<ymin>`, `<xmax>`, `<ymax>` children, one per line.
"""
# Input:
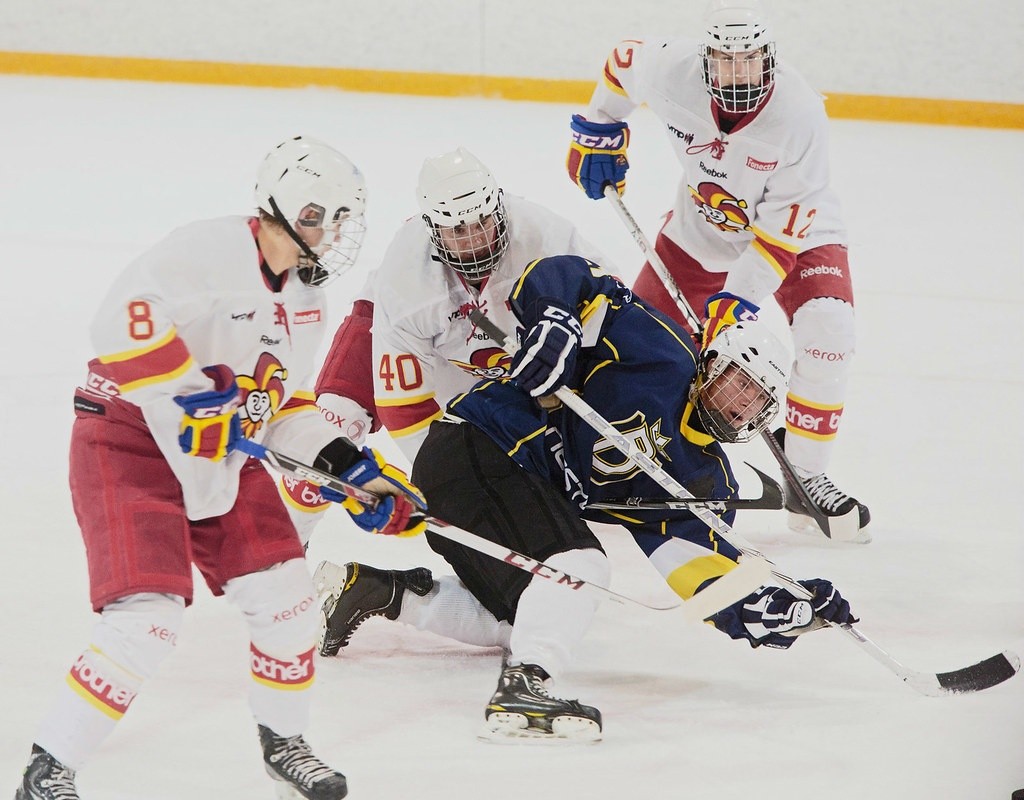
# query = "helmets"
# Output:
<box><xmin>416</xmin><ymin>150</ymin><xmax>513</xmax><ymax>282</ymax></box>
<box><xmin>250</xmin><ymin>137</ymin><xmax>368</xmax><ymax>289</ymax></box>
<box><xmin>690</xmin><ymin>321</ymin><xmax>796</xmax><ymax>444</ymax></box>
<box><xmin>698</xmin><ymin>5</ymin><xmax>778</xmax><ymax>113</ymax></box>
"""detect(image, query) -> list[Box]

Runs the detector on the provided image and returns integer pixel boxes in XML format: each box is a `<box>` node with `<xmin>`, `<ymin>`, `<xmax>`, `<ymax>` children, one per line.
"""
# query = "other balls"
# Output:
<box><xmin>1012</xmin><ymin>789</ymin><xmax>1024</xmax><ymax>800</ymax></box>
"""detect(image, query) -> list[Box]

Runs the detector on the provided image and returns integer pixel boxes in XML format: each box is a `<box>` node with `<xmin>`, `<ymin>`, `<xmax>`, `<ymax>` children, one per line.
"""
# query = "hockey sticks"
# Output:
<box><xmin>74</xmin><ymin>387</ymin><xmax>771</xmax><ymax>622</ymax></box>
<box><xmin>602</xmin><ymin>183</ymin><xmax>870</xmax><ymax>538</ymax></box>
<box><xmin>464</xmin><ymin>304</ymin><xmax>1020</xmax><ymax>697</ymax></box>
<box><xmin>585</xmin><ymin>460</ymin><xmax>786</xmax><ymax>510</ymax></box>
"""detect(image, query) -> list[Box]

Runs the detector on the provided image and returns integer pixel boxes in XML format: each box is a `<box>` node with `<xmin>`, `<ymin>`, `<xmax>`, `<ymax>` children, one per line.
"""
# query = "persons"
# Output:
<box><xmin>13</xmin><ymin>134</ymin><xmax>426</xmax><ymax>799</ymax></box>
<box><xmin>568</xmin><ymin>9</ymin><xmax>874</xmax><ymax>543</ymax></box>
<box><xmin>276</xmin><ymin>149</ymin><xmax>618</xmax><ymax>553</ymax></box>
<box><xmin>312</xmin><ymin>253</ymin><xmax>859</xmax><ymax>743</ymax></box>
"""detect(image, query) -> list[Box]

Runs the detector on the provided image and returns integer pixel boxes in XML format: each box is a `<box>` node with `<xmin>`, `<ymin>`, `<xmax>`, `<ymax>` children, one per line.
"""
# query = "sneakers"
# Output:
<box><xmin>312</xmin><ymin>560</ymin><xmax>433</xmax><ymax>657</ymax></box>
<box><xmin>784</xmin><ymin>465</ymin><xmax>872</xmax><ymax>544</ymax></box>
<box><xmin>15</xmin><ymin>743</ymin><xmax>81</xmax><ymax>800</ymax></box>
<box><xmin>257</xmin><ymin>723</ymin><xmax>348</xmax><ymax>800</ymax></box>
<box><xmin>476</xmin><ymin>648</ymin><xmax>605</xmax><ymax>748</ymax></box>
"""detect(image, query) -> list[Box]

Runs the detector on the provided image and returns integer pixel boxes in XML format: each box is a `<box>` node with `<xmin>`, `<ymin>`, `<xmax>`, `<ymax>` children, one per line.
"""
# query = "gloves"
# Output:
<box><xmin>743</xmin><ymin>578</ymin><xmax>860</xmax><ymax>649</ymax></box>
<box><xmin>702</xmin><ymin>292</ymin><xmax>760</xmax><ymax>349</ymax></box>
<box><xmin>319</xmin><ymin>446</ymin><xmax>428</xmax><ymax>538</ymax></box>
<box><xmin>508</xmin><ymin>298</ymin><xmax>584</xmax><ymax>398</ymax></box>
<box><xmin>566</xmin><ymin>114</ymin><xmax>630</xmax><ymax>200</ymax></box>
<box><xmin>173</xmin><ymin>363</ymin><xmax>241</xmax><ymax>463</ymax></box>
